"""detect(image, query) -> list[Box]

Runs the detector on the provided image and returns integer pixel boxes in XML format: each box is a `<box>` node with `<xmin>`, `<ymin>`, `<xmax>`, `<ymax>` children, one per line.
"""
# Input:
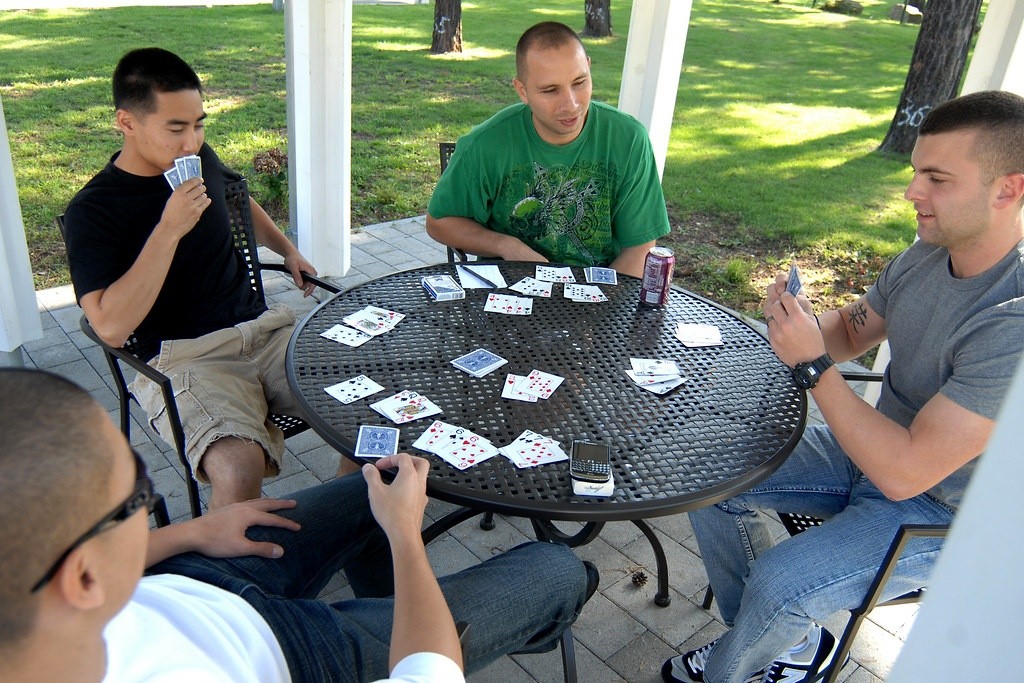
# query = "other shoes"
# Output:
<box><xmin>581</xmin><ymin>560</ymin><xmax>599</xmax><ymax>605</ymax></box>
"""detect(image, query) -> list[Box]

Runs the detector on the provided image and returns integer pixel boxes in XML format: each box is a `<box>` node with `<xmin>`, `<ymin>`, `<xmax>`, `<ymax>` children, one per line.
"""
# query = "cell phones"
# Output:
<box><xmin>570</xmin><ymin>439</ymin><xmax>611</xmax><ymax>483</ymax></box>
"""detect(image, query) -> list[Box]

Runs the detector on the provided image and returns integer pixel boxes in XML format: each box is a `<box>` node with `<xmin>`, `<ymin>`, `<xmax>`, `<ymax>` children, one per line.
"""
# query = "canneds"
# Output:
<box><xmin>639</xmin><ymin>246</ymin><xmax>675</xmax><ymax>306</ymax></box>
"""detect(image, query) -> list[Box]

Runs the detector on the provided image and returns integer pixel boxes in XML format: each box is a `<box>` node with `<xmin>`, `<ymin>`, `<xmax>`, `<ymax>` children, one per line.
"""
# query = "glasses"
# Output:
<box><xmin>27</xmin><ymin>447</ymin><xmax>155</xmax><ymax>595</ymax></box>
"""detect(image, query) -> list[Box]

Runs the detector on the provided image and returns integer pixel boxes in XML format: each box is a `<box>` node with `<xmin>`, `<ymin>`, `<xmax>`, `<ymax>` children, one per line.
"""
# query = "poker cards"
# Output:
<box><xmin>164</xmin><ymin>155</ymin><xmax>203</xmax><ymax>190</ymax></box>
<box><xmin>321</xmin><ymin>263</ymin><xmax>725</xmax><ymax>467</ymax></box>
<box><xmin>785</xmin><ymin>265</ymin><xmax>805</xmax><ymax>298</ymax></box>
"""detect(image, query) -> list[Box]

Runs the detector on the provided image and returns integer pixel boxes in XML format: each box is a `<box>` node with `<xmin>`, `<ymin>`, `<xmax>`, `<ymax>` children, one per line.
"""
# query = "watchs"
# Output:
<box><xmin>792</xmin><ymin>353</ymin><xmax>835</xmax><ymax>390</ymax></box>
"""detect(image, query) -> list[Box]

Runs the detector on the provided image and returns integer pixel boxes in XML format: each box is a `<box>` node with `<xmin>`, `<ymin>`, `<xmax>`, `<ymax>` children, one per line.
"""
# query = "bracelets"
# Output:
<box><xmin>813</xmin><ymin>313</ymin><xmax>824</xmax><ymax>329</ymax></box>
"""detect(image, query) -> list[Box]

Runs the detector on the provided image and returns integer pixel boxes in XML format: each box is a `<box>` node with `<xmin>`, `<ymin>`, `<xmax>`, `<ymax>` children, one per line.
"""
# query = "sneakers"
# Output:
<box><xmin>743</xmin><ymin>622</ymin><xmax>850</xmax><ymax>683</ymax></box>
<box><xmin>661</xmin><ymin>635</ymin><xmax>728</xmax><ymax>683</ymax></box>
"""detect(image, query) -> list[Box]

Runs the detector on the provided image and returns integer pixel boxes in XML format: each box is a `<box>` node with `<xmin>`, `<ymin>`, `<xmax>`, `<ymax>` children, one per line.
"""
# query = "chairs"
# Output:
<box><xmin>432</xmin><ymin>143</ymin><xmax>617</xmax><ymax>415</ymax></box>
<box><xmin>57</xmin><ymin>178</ymin><xmax>344</xmax><ymax>517</ymax></box>
<box><xmin>704</xmin><ymin>369</ymin><xmax>951</xmax><ymax>683</ymax></box>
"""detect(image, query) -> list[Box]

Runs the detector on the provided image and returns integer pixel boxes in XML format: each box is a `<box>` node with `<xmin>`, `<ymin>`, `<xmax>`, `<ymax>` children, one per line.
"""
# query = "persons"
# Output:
<box><xmin>426</xmin><ymin>22</ymin><xmax>670</xmax><ymax>277</ymax></box>
<box><xmin>659</xmin><ymin>91</ymin><xmax>1024</xmax><ymax>683</ymax></box>
<box><xmin>63</xmin><ymin>48</ymin><xmax>365</xmax><ymax>515</ymax></box>
<box><xmin>0</xmin><ymin>367</ymin><xmax>599</xmax><ymax>682</ymax></box>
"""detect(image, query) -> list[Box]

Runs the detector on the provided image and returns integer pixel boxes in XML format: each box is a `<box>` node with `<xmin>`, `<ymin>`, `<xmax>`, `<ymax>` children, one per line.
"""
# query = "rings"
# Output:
<box><xmin>766</xmin><ymin>316</ymin><xmax>775</xmax><ymax>327</ymax></box>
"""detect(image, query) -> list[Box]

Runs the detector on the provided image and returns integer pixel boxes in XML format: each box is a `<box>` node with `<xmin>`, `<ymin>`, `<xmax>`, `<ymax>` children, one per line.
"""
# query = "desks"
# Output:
<box><xmin>287</xmin><ymin>262</ymin><xmax>807</xmax><ymax>683</ymax></box>
